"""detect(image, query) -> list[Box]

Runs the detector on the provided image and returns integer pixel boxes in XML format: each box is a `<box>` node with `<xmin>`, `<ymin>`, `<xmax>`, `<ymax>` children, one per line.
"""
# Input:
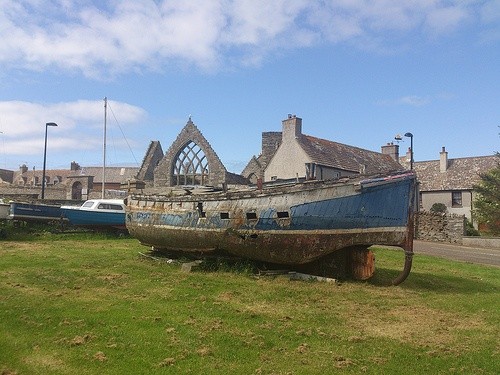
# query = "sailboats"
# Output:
<box><xmin>58</xmin><ymin>96</ymin><xmax>143</xmax><ymax>227</ymax></box>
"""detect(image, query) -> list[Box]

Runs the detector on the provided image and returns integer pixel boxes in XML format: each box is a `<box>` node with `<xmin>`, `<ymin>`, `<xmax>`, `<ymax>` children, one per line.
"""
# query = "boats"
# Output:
<box><xmin>125</xmin><ymin>166</ymin><xmax>417</xmax><ymax>289</ymax></box>
<box><xmin>6</xmin><ymin>202</ymin><xmax>67</xmax><ymax>225</ymax></box>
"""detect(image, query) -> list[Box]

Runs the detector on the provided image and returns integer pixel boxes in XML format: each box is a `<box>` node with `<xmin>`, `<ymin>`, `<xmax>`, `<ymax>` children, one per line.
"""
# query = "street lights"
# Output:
<box><xmin>41</xmin><ymin>123</ymin><xmax>58</xmax><ymax>199</ymax></box>
<box><xmin>403</xmin><ymin>133</ymin><xmax>413</xmax><ymax>171</ymax></box>
